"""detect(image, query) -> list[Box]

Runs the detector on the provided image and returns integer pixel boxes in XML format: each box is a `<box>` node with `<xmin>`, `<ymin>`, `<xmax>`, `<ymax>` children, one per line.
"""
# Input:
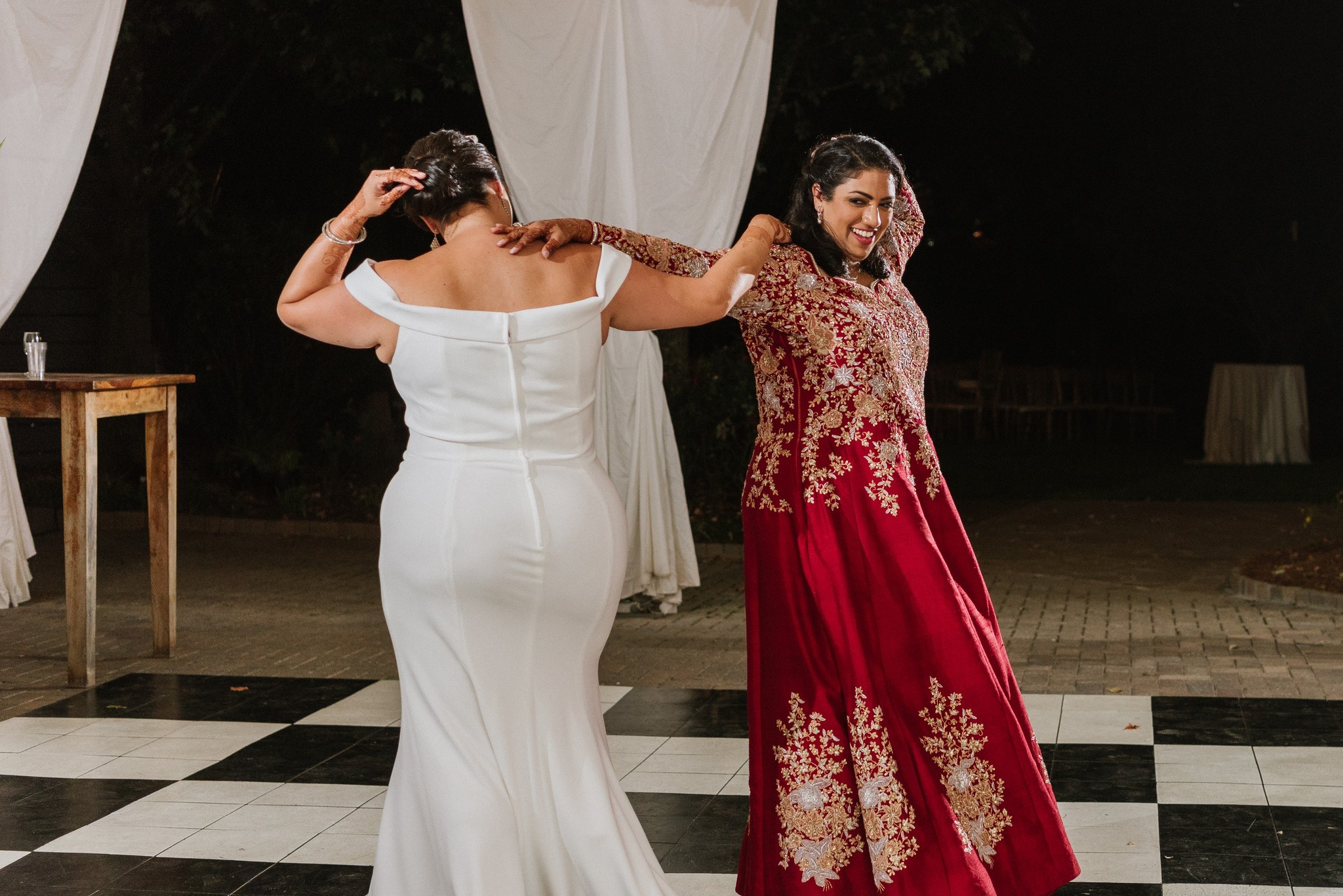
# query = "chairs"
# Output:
<box><xmin>925</xmin><ymin>349</ymin><xmax>1173</xmax><ymax>445</ymax></box>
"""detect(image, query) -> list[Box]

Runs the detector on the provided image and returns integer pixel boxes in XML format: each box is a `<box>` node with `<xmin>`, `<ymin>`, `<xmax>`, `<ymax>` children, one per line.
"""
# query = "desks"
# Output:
<box><xmin>1203</xmin><ymin>361</ymin><xmax>1313</xmax><ymax>466</ymax></box>
<box><xmin>0</xmin><ymin>372</ymin><xmax>197</xmax><ymax>690</ymax></box>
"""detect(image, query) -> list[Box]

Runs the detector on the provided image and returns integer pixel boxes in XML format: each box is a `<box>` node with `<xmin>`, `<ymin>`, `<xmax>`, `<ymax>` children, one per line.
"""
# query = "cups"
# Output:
<box><xmin>27</xmin><ymin>341</ymin><xmax>47</xmax><ymax>378</ymax></box>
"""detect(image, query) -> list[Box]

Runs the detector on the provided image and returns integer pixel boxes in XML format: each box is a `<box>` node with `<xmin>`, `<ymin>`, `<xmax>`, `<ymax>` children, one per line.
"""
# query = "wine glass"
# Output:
<box><xmin>23</xmin><ymin>332</ymin><xmax>41</xmax><ymax>378</ymax></box>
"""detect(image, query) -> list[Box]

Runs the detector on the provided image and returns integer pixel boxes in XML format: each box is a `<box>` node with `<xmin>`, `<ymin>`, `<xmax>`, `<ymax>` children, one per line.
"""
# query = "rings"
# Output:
<box><xmin>784</xmin><ymin>226</ymin><xmax>792</xmax><ymax>236</ymax></box>
<box><xmin>389</xmin><ymin>165</ymin><xmax>396</xmax><ymax>171</ymax></box>
<box><xmin>512</xmin><ymin>221</ymin><xmax>524</xmax><ymax>227</ymax></box>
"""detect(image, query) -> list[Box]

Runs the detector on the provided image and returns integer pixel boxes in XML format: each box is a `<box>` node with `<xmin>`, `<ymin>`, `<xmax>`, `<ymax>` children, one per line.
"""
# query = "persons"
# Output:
<box><xmin>493</xmin><ymin>129</ymin><xmax>1082</xmax><ymax>896</ymax></box>
<box><xmin>271</xmin><ymin>125</ymin><xmax>795</xmax><ymax>895</ymax></box>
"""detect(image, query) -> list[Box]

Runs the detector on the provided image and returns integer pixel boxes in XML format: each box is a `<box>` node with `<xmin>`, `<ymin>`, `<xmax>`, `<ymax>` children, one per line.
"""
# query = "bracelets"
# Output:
<box><xmin>322</xmin><ymin>221</ymin><xmax>364</xmax><ymax>244</ymax></box>
<box><xmin>584</xmin><ymin>218</ymin><xmax>598</xmax><ymax>245</ymax></box>
<box><xmin>326</xmin><ymin>217</ymin><xmax>367</xmax><ymax>243</ymax></box>
